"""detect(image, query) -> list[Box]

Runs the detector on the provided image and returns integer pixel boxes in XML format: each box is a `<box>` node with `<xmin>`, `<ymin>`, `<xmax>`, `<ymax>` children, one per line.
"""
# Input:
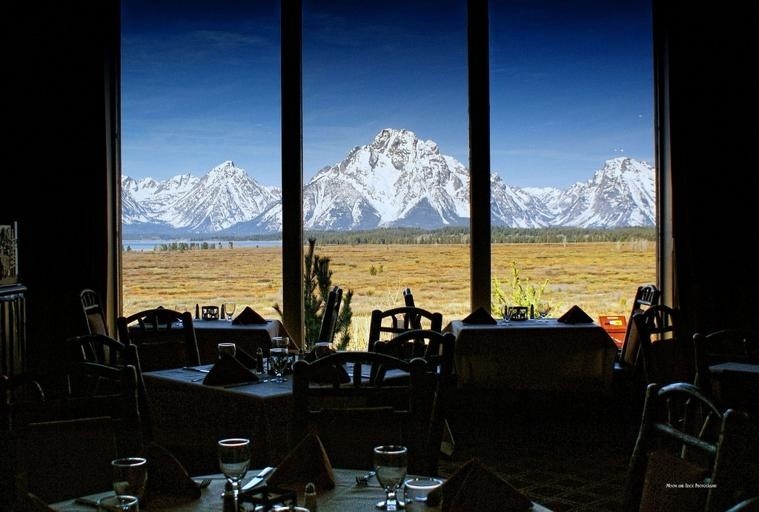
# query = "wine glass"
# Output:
<box><xmin>218</xmin><ymin>336</ymin><xmax>337</xmax><ymax>388</ymax></box>
<box><xmin>97</xmin><ymin>435</ymin><xmax>445</xmax><ymax>512</ymax></box>
<box><xmin>175</xmin><ymin>306</ymin><xmax>188</xmax><ymax>326</ymax></box>
<box><xmin>224</xmin><ymin>303</ymin><xmax>235</xmax><ymax>324</ymax></box>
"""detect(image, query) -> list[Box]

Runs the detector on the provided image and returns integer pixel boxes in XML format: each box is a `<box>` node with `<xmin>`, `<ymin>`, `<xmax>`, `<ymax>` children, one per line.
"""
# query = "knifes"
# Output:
<box><xmin>183</xmin><ymin>366</ymin><xmax>210</xmax><ymax>373</ymax></box>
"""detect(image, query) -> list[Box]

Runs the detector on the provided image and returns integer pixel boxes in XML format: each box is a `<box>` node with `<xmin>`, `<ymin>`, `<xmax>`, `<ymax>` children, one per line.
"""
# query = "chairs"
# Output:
<box><xmin>0</xmin><ymin>285</ymin><xmax>759</xmax><ymax>512</ymax></box>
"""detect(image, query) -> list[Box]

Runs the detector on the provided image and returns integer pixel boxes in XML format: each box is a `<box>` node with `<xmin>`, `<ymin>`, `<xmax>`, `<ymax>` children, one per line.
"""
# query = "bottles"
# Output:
<box><xmin>530</xmin><ymin>304</ymin><xmax>534</xmax><ymax>319</ymax></box>
<box><xmin>220</xmin><ymin>303</ymin><xmax>225</xmax><ymax>320</ymax></box>
<box><xmin>194</xmin><ymin>304</ymin><xmax>200</xmax><ymax>320</ymax></box>
